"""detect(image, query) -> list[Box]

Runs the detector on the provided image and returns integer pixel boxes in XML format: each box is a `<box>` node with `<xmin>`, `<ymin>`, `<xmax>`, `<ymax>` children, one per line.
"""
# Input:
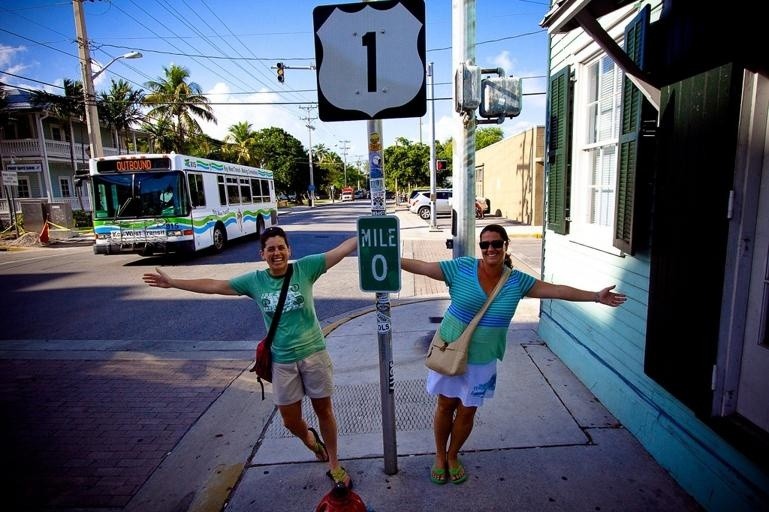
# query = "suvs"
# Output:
<box><xmin>406</xmin><ymin>188</ymin><xmax>491</xmax><ymax>219</ymax></box>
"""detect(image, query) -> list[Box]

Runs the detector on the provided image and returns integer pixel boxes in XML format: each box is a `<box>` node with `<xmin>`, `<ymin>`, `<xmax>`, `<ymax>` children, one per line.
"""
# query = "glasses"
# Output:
<box><xmin>480</xmin><ymin>240</ymin><xmax>506</xmax><ymax>249</ymax></box>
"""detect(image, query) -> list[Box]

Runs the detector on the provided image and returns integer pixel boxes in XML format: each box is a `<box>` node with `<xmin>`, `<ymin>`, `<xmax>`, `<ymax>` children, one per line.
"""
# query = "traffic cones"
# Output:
<box><xmin>37</xmin><ymin>222</ymin><xmax>49</xmax><ymax>245</ymax></box>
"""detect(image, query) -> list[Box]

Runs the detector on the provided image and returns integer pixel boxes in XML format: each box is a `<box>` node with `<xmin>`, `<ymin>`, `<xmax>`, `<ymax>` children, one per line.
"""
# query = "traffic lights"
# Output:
<box><xmin>436</xmin><ymin>160</ymin><xmax>448</xmax><ymax>171</ymax></box>
<box><xmin>276</xmin><ymin>61</ymin><xmax>285</xmax><ymax>84</ymax></box>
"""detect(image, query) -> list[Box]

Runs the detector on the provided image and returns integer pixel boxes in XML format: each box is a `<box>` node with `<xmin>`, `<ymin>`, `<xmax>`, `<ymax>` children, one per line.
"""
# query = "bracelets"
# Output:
<box><xmin>594</xmin><ymin>291</ymin><xmax>600</xmax><ymax>302</ymax></box>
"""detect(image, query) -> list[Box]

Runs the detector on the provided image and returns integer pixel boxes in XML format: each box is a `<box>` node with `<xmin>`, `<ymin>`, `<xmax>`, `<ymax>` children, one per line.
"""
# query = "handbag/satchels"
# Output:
<box><xmin>424</xmin><ymin>333</ymin><xmax>469</xmax><ymax>376</ymax></box>
<box><xmin>256</xmin><ymin>340</ymin><xmax>271</xmax><ymax>382</ymax></box>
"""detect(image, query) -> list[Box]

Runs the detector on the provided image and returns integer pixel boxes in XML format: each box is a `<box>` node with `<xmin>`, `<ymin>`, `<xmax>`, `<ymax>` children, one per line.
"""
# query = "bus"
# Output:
<box><xmin>87</xmin><ymin>151</ymin><xmax>279</xmax><ymax>266</ymax></box>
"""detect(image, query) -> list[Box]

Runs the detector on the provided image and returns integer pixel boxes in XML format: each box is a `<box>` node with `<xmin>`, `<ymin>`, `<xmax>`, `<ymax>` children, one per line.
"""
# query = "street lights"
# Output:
<box><xmin>78</xmin><ymin>50</ymin><xmax>143</xmax><ymax>158</ymax></box>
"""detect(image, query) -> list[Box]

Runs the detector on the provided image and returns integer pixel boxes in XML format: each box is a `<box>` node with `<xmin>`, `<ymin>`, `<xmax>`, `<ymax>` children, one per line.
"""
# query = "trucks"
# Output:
<box><xmin>341</xmin><ymin>188</ymin><xmax>355</xmax><ymax>202</ymax></box>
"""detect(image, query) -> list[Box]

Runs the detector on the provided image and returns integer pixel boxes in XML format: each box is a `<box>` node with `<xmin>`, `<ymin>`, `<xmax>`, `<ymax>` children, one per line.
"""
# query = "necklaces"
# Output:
<box><xmin>482</xmin><ymin>262</ymin><xmax>504</xmax><ymax>294</ymax></box>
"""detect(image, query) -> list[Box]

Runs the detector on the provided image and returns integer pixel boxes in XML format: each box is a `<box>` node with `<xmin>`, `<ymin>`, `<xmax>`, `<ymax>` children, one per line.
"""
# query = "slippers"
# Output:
<box><xmin>326</xmin><ymin>466</ymin><xmax>353</xmax><ymax>490</ymax></box>
<box><xmin>431</xmin><ymin>459</ymin><xmax>467</xmax><ymax>484</ymax></box>
<box><xmin>308</xmin><ymin>427</ymin><xmax>329</xmax><ymax>462</ymax></box>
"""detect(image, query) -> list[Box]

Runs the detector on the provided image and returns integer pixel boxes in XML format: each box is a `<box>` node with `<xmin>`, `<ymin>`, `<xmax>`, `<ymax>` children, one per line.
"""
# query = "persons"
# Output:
<box><xmin>399</xmin><ymin>225</ymin><xmax>627</xmax><ymax>485</ymax></box>
<box><xmin>278</xmin><ymin>190</ymin><xmax>304</xmax><ymax>205</ymax></box>
<box><xmin>474</xmin><ymin>199</ymin><xmax>484</xmax><ymax>219</ymax></box>
<box><xmin>143</xmin><ymin>227</ymin><xmax>359</xmax><ymax>492</ymax></box>
